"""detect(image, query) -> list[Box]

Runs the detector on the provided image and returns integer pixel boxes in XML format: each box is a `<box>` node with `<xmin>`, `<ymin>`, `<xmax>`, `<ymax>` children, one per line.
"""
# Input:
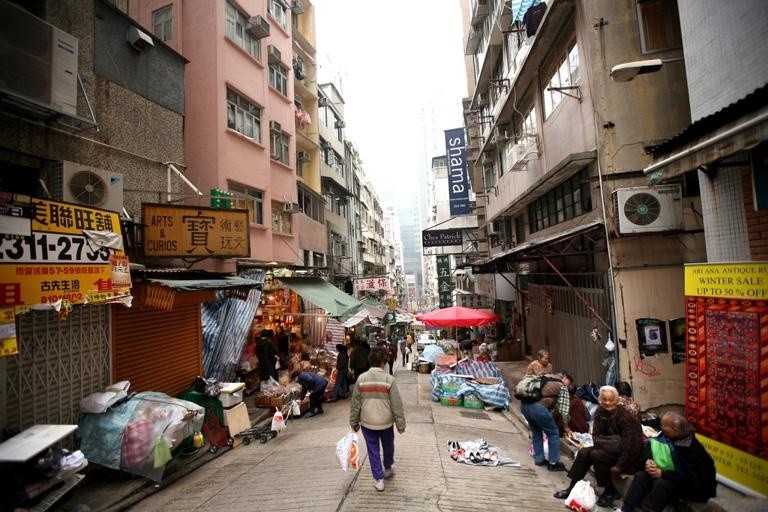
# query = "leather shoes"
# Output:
<box><xmin>553</xmin><ymin>491</ymin><xmax>571</xmax><ymax>499</ymax></box>
<box><xmin>597</xmin><ymin>488</ymin><xmax>621</xmax><ymax>508</ymax></box>
<box><xmin>455</xmin><ymin>440</ymin><xmax>465</xmax><ymax>459</ymax></box>
<box><xmin>449</xmin><ymin>441</ymin><xmax>498</xmax><ymax>466</ymax></box>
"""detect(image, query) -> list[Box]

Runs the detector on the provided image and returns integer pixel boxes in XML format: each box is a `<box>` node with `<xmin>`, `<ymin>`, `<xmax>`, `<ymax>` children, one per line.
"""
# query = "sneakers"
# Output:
<box><xmin>547</xmin><ymin>461</ymin><xmax>566</xmax><ymax>472</ymax></box>
<box><xmin>372</xmin><ymin>479</ymin><xmax>385</xmax><ymax>492</ymax></box>
<box><xmin>384</xmin><ymin>464</ymin><xmax>393</xmax><ymax>478</ymax></box>
<box><xmin>535</xmin><ymin>459</ymin><xmax>549</xmax><ymax>466</ymax></box>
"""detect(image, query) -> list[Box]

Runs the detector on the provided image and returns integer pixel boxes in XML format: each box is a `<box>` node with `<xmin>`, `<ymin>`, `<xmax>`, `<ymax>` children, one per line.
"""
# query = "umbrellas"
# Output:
<box><xmin>415</xmin><ymin>306</ymin><xmax>499</xmax><ymax>366</ymax></box>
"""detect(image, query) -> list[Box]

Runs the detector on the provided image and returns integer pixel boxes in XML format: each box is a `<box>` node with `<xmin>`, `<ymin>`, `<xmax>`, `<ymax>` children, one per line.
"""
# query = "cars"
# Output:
<box><xmin>417</xmin><ymin>334</ymin><xmax>438</xmax><ymax>351</ymax></box>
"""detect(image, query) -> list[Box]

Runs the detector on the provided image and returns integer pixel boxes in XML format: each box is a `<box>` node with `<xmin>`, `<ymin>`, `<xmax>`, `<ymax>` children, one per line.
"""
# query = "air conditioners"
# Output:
<box><xmin>611</xmin><ymin>183</ymin><xmax>688</xmax><ymax>236</ymax></box>
<box><xmin>284</xmin><ymin>202</ymin><xmax>302</xmax><ymax>212</ymax></box>
<box><xmin>60</xmin><ymin>158</ymin><xmax>126</xmax><ymax>213</ymax></box>
<box><xmin>504</xmin><ymin>144</ymin><xmax>526</xmax><ymax>170</ymax></box>
<box><xmin>298</xmin><ymin>150</ymin><xmax>311</xmax><ymax>162</ymax></box>
<box><xmin>267</xmin><ymin>44</ymin><xmax>281</xmax><ymax>64</ymax></box>
<box><xmin>0</xmin><ymin>0</ymin><xmax>79</xmax><ymax>118</ymax></box>
<box><xmin>268</xmin><ymin>119</ymin><xmax>280</xmax><ymax>135</ymax></box>
<box><xmin>475</xmin><ymin>91</ymin><xmax>487</xmax><ymax>106</ymax></box>
<box><xmin>245</xmin><ymin>14</ymin><xmax>270</xmax><ymax>40</ymax></box>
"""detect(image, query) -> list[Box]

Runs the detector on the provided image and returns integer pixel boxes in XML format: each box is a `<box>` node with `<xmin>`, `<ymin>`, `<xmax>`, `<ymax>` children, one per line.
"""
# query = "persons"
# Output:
<box><xmin>290</xmin><ymin>370</ymin><xmax>328</xmax><ymax>418</ymax></box>
<box><xmin>614</xmin><ymin>381</ymin><xmax>642</xmax><ymax>422</ymax></box>
<box><xmin>386</xmin><ymin>347</ymin><xmax>406</xmax><ymax>375</ymax></box>
<box><xmin>255</xmin><ymin>326</ymin><xmax>290</xmax><ymax>382</ymax></box>
<box><xmin>554</xmin><ymin>385</ymin><xmax>648</xmax><ymax>507</ymax></box>
<box><xmin>375</xmin><ymin>330</ymin><xmax>406</xmax><ymax>347</ymax></box>
<box><xmin>350</xmin><ymin>347</ymin><xmax>406</xmax><ymax>491</ymax></box>
<box><xmin>326</xmin><ymin>344</ymin><xmax>348</xmax><ymax>403</ymax></box>
<box><xmin>348</xmin><ymin>337</ymin><xmax>370</xmax><ymax>382</ymax></box>
<box><xmin>614</xmin><ymin>412</ymin><xmax>716</xmax><ymax>512</ymax></box>
<box><xmin>458</xmin><ymin>340</ymin><xmax>477</xmax><ymax>359</ymax></box>
<box><xmin>406</xmin><ymin>331</ymin><xmax>416</xmax><ymax>363</ymax></box>
<box><xmin>521</xmin><ymin>371</ymin><xmax>573</xmax><ymax>470</ymax></box>
<box><xmin>551</xmin><ymin>383</ymin><xmax>591</xmax><ymax>437</ymax></box>
<box><xmin>341</xmin><ymin>346</ymin><xmax>349</xmax><ymax>394</ymax></box>
<box><xmin>525</xmin><ymin>349</ymin><xmax>553</xmax><ymax>377</ymax></box>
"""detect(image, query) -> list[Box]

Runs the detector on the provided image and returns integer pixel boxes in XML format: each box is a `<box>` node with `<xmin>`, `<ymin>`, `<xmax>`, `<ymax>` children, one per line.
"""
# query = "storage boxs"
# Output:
<box><xmin>411</xmin><ymin>360</ymin><xmax>433</xmax><ymax>374</ymax></box>
<box><xmin>212</xmin><ymin>382</ymin><xmax>245</xmax><ymax>408</ymax></box>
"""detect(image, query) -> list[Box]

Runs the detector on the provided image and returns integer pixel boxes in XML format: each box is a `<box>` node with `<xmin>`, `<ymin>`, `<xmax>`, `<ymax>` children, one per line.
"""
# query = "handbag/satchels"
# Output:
<box><xmin>646</xmin><ymin>437</ymin><xmax>682</xmax><ymax>481</ymax></box>
<box><xmin>596</xmin><ymin>434</ymin><xmax>625</xmax><ymax>456</ymax></box>
<box><xmin>335</xmin><ymin>430</ymin><xmax>353</xmax><ymax>473</ymax></box>
<box><xmin>351</xmin><ymin>433</ymin><xmax>359</xmax><ymax>473</ymax></box>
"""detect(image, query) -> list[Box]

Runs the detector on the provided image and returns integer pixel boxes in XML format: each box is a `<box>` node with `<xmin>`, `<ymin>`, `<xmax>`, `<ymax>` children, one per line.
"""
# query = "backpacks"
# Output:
<box><xmin>513</xmin><ymin>373</ymin><xmax>564</xmax><ymax>404</ymax></box>
